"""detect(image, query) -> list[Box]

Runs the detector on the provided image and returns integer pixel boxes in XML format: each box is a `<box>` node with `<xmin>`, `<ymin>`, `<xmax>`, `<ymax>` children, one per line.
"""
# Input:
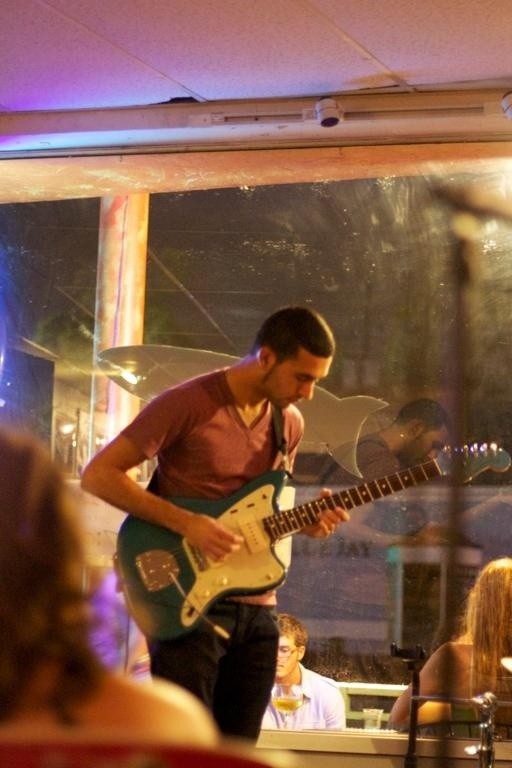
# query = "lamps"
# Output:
<box><xmin>501</xmin><ymin>90</ymin><xmax>512</xmax><ymax>116</ymax></box>
<box><xmin>316</xmin><ymin>96</ymin><xmax>340</xmax><ymax>128</ymax></box>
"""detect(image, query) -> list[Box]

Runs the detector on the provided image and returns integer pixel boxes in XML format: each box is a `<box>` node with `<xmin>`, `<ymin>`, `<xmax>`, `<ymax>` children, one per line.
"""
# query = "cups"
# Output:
<box><xmin>362</xmin><ymin>707</ymin><xmax>385</xmax><ymax>730</ymax></box>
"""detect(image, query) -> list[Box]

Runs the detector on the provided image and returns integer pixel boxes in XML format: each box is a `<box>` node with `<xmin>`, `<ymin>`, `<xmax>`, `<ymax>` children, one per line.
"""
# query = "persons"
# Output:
<box><xmin>78</xmin><ymin>304</ymin><xmax>351</xmax><ymax>748</ymax></box>
<box><xmin>299</xmin><ymin>397</ymin><xmax>454</xmax><ymax>621</ymax></box>
<box><xmin>259</xmin><ymin>612</ymin><xmax>346</xmax><ymax>733</ymax></box>
<box><xmin>386</xmin><ymin>553</ymin><xmax>512</xmax><ymax>742</ymax></box>
<box><xmin>0</xmin><ymin>420</ymin><xmax>224</xmax><ymax>748</ymax></box>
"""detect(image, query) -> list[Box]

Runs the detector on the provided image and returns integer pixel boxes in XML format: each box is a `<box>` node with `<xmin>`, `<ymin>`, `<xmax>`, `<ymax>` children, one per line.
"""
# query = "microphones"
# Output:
<box><xmin>167</xmin><ymin>570</ymin><xmax>231</xmax><ymax>640</ymax></box>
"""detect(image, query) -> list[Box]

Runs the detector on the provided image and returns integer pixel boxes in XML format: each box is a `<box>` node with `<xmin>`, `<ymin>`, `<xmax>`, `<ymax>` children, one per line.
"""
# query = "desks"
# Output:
<box><xmin>336</xmin><ymin>682</ymin><xmax>408</xmax><ymax>730</ymax></box>
<box><xmin>256</xmin><ymin>728</ymin><xmax>512</xmax><ymax>768</ymax></box>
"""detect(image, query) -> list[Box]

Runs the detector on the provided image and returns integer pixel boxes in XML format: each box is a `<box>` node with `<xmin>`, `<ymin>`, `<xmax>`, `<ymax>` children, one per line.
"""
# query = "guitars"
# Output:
<box><xmin>113</xmin><ymin>441</ymin><xmax>511</xmax><ymax>639</ymax></box>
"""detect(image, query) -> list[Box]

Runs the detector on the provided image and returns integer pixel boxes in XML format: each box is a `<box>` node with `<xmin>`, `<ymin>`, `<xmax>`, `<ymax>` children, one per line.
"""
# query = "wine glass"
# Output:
<box><xmin>272</xmin><ymin>682</ymin><xmax>304</xmax><ymax>731</ymax></box>
